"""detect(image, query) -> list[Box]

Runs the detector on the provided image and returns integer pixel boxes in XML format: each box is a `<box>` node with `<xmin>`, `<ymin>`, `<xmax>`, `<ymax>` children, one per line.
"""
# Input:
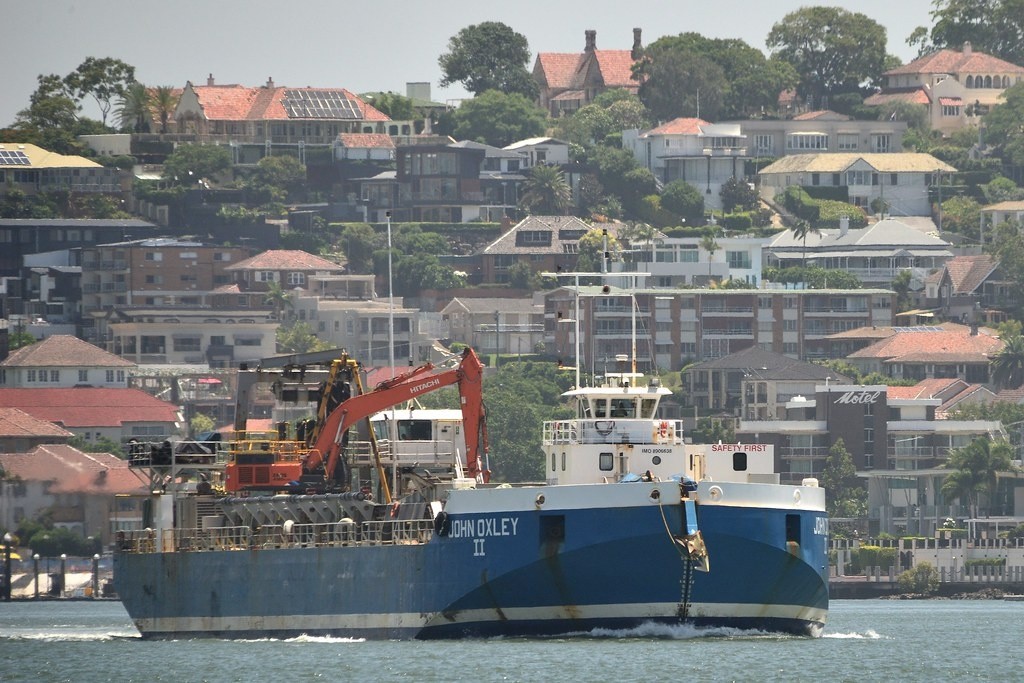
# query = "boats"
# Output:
<box><xmin>108</xmin><ymin>212</ymin><xmax>831</xmax><ymax>638</ymax></box>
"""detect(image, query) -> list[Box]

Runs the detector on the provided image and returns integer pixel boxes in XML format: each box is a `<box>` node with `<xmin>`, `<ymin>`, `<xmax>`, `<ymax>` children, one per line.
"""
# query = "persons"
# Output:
<box><xmin>614</xmin><ymin>401</ymin><xmax>627</xmax><ymax>416</ymax></box>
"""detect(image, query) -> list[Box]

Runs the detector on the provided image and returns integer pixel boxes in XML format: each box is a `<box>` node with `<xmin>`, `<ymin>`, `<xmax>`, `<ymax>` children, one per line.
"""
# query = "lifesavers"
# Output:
<box><xmin>390</xmin><ymin>502</ymin><xmax>400</xmax><ymax>517</ymax></box>
<box><xmin>434</xmin><ymin>512</ymin><xmax>450</xmax><ymax>537</ymax></box>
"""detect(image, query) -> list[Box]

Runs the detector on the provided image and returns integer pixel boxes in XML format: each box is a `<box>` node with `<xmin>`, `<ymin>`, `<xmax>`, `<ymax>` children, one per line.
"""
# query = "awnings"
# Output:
<box><xmin>196</xmin><ymin>378</ymin><xmax>221</xmax><ymax>384</ymax></box>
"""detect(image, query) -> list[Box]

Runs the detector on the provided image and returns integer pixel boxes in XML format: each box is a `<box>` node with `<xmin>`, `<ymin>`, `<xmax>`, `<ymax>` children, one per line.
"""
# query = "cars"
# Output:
<box><xmin>0</xmin><ymin>544</ymin><xmax>22</xmax><ymax>567</ymax></box>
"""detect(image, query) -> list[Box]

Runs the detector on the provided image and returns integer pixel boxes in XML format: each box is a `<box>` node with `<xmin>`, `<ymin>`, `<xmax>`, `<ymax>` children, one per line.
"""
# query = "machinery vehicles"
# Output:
<box><xmin>223</xmin><ymin>345</ymin><xmax>493</xmax><ymax>495</ymax></box>
